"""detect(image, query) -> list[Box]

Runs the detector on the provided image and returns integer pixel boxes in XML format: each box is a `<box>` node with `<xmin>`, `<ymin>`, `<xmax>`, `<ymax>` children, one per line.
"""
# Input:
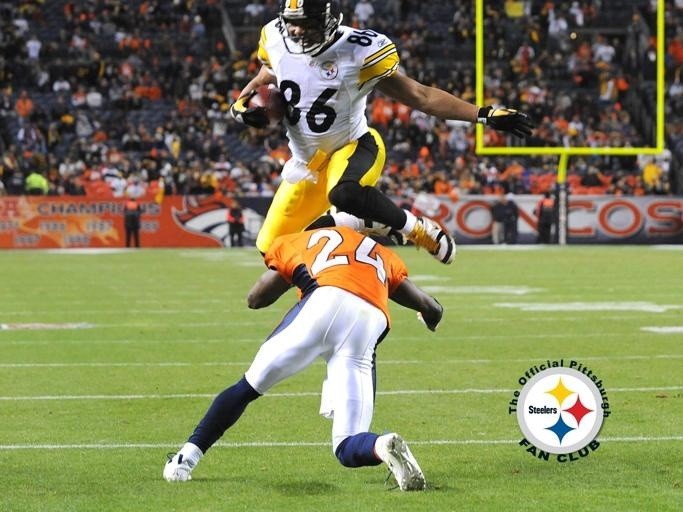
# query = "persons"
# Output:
<box><xmin>226</xmin><ymin>200</ymin><xmax>245</xmax><ymax>247</ymax></box>
<box><xmin>1</xmin><ymin>1</ymin><xmax>683</xmax><ymax>199</ymax></box>
<box><xmin>397</xmin><ymin>195</ymin><xmax>414</xmax><ymax>214</ymax></box>
<box><xmin>160</xmin><ymin>224</ymin><xmax>447</xmax><ymax>492</ymax></box>
<box><xmin>500</xmin><ymin>191</ymin><xmax>521</xmax><ymax>244</ymax></box>
<box><xmin>121</xmin><ymin>196</ymin><xmax>146</xmax><ymax>247</ymax></box>
<box><xmin>533</xmin><ymin>190</ymin><xmax>555</xmax><ymax>244</ymax></box>
<box><xmin>228</xmin><ymin>0</ymin><xmax>540</xmax><ymax>267</ymax></box>
<box><xmin>487</xmin><ymin>194</ymin><xmax>505</xmax><ymax>244</ymax></box>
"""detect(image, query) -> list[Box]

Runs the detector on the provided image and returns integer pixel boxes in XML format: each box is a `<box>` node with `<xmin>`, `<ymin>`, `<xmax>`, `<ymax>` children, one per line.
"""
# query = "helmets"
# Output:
<box><xmin>278</xmin><ymin>0</ymin><xmax>343</xmax><ymax>40</ymax></box>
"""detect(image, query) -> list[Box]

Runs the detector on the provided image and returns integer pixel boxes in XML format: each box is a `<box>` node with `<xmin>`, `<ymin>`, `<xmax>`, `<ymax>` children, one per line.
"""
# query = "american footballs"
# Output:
<box><xmin>248</xmin><ymin>84</ymin><xmax>285</xmax><ymax>127</ymax></box>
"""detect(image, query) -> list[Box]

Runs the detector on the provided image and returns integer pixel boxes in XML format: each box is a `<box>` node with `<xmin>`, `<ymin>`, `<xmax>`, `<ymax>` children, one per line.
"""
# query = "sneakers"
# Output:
<box><xmin>162</xmin><ymin>451</ymin><xmax>194</xmax><ymax>484</ymax></box>
<box><xmin>373</xmin><ymin>430</ymin><xmax>427</xmax><ymax>494</ymax></box>
<box><xmin>403</xmin><ymin>210</ymin><xmax>458</xmax><ymax>269</ymax></box>
<box><xmin>363</xmin><ymin>219</ymin><xmax>408</xmax><ymax>249</ymax></box>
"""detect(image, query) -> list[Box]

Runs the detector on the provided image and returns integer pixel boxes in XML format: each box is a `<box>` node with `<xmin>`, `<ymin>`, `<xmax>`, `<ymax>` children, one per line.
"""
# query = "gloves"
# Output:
<box><xmin>228</xmin><ymin>89</ymin><xmax>272</xmax><ymax>132</ymax></box>
<box><xmin>477</xmin><ymin>104</ymin><xmax>535</xmax><ymax>139</ymax></box>
